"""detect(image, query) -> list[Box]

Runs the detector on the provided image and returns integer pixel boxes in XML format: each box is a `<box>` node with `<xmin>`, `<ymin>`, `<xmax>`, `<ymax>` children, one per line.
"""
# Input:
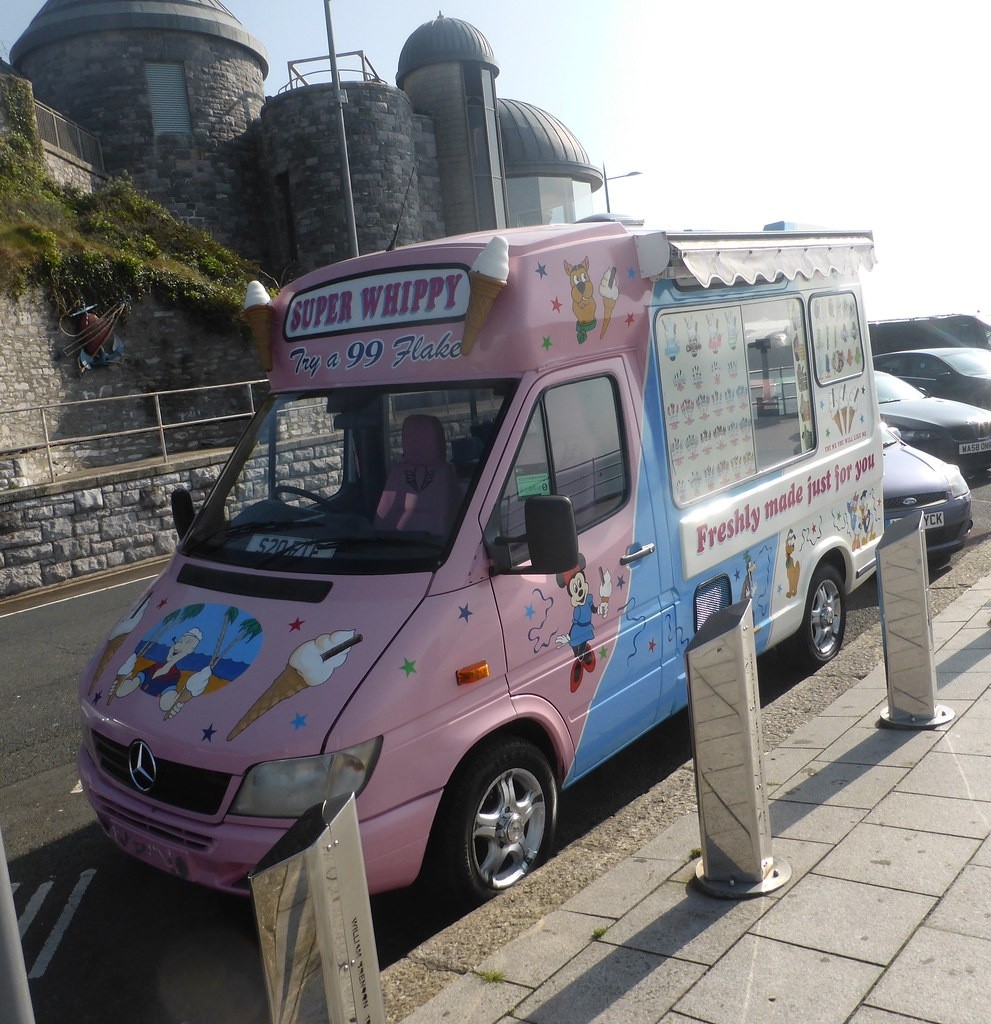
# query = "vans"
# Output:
<box><xmin>75</xmin><ymin>213</ymin><xmax>886</xmax><ymax>912</ymax></box>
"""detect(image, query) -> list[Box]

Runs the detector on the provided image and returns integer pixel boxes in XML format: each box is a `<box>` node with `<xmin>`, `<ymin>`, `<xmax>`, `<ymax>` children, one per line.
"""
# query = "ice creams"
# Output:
<box><xmin>460</xmin><ymin>236</ymin><xmax>509</xmax><ymax>356</ymax></box>
<box><xmin>244</xmin><ymin>280</ymin><xmax>275</xmax><ymax>371</ymax></box>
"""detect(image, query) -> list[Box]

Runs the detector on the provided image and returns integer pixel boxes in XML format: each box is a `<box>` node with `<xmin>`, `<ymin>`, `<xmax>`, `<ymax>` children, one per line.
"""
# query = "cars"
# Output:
<box><xmin>874</xmin><ymin>368</ymin><xmax>991</xmax><ymax>474</ymax></box>
<box><xmin>871</xmin><ymin>346</ymin><xmax>991</xmax><ymax>411</ymax></box>
<box><xmin>880</xmin><ymin>421</ymin><xmax>975</xmax><ymax>556</ymax></box>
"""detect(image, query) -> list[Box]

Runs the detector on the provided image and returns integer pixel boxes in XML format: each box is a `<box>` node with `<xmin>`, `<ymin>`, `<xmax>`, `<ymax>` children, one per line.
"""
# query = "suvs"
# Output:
<box><xmin>866</xmin><ymin>313</ymin><xmax>991</xmax><ymax>357</ymax></box>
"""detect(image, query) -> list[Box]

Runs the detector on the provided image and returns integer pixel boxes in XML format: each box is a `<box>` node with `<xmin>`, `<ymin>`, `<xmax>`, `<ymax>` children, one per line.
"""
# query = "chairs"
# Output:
<box><xmin>379</xmin><ymin>417</ymin><xmax>450</xmax><ymax>532</ymax></box>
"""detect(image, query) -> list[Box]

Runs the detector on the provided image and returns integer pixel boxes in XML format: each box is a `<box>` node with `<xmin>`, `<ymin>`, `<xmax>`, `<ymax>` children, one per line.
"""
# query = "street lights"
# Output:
<box><xmin>602</xmin><ymin>160</ymin><xmax>643</xmax><ymax>212</ymax></box>
